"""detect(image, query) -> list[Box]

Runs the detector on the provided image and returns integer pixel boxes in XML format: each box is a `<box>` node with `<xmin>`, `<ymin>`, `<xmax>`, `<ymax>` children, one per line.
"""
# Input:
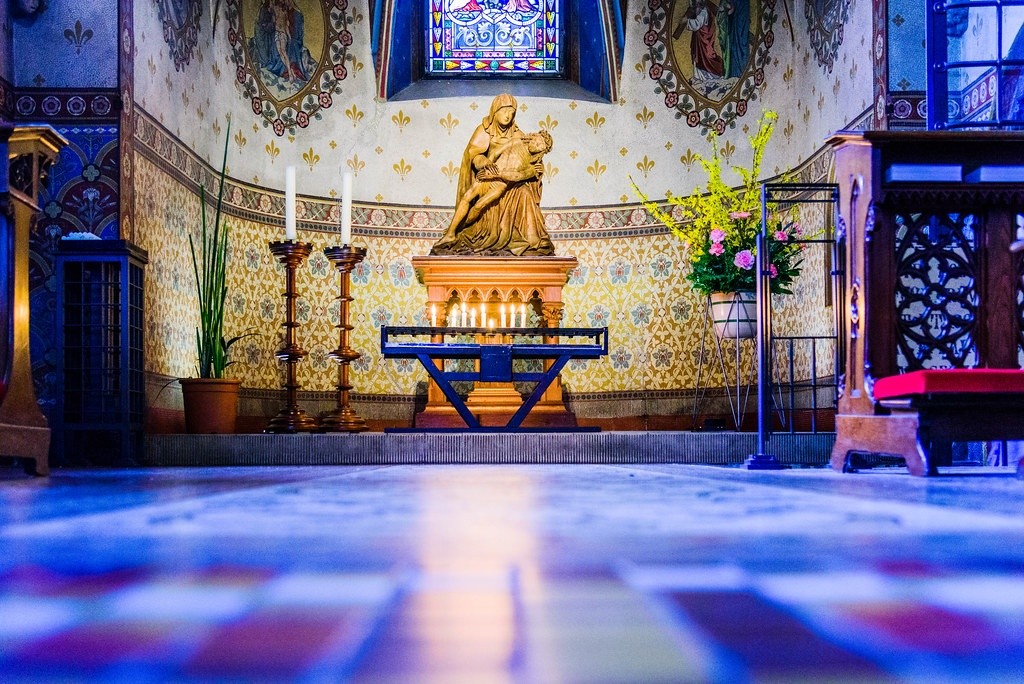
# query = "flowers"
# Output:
<box><xmin>626</xmin><ymin>107</ymin><xmax>835</xmax><ymax>295</ymax></box>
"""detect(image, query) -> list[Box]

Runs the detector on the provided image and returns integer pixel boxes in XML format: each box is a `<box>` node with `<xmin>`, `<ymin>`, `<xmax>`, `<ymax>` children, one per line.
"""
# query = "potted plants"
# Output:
<box><xmin>178</xmin><ymin>116</ymin><xmax>263</xmax><ymax>432</ymax></box>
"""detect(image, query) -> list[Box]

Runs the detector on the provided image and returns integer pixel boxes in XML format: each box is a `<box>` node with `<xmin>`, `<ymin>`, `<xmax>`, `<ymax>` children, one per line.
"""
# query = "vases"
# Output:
<box><xmin>710</xmin><ymin>289</ymin><xmax>756</xmax><ymax>340</ymax></box>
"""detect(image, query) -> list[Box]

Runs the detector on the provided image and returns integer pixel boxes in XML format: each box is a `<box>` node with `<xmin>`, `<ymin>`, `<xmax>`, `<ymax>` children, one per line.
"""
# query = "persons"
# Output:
<box><xmin>433</xmin><ymin>129</ymin><xmax>552</xmax><ymax>247</ymax></box>
<box><xmin>455</xmin><ymin>93</ymin><xmax>555</xmax><ymax>257</ymax></box>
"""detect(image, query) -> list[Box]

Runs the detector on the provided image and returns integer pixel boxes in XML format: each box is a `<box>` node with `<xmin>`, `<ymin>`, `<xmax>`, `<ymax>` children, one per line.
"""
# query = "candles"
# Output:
<box><xmin>462</xmin><ymin>303</ymin><xmax>467</xmax><ymax>327</ymax></box>
<box><xmin>510</xmin><ymin>305</ymin><xmax>516</xmax><ymax>327</ymax></box>
<box><xmin>341</xmin><ymin>173</ymin><xmax>352</xmax><ymax>244</ymax></box>
<box><xmin>285</xmin><ymin>166</ymin><xmax>297</xmax><ymax>238</ymax></box>
<box><xmin>500</xmin><ymin>303</ymin><xmax>507</xmax><ymax>328</ymax></box>
<box><xmin>470</xmin><ymin>308</ymin><xmax>476</xmax><ymax>326</ymax></box>
<box><xmin>480</xmin><ymin>304</ymin><xmax>486</xmax><ymax>327</ymax></box>
<box><xmin>451</xmin><ymin>309</ymin><xmax>456</xmax><ymax>328</ymax></box>
<box><xmin>431</xmin><ymin>304</ymin><xmax>436</xmax><ymax>327</ymax></box>
<box><xmin>520</xmin><ymin>303</ymin><xmax>528</xmax><ymax>328</ymax></box>
